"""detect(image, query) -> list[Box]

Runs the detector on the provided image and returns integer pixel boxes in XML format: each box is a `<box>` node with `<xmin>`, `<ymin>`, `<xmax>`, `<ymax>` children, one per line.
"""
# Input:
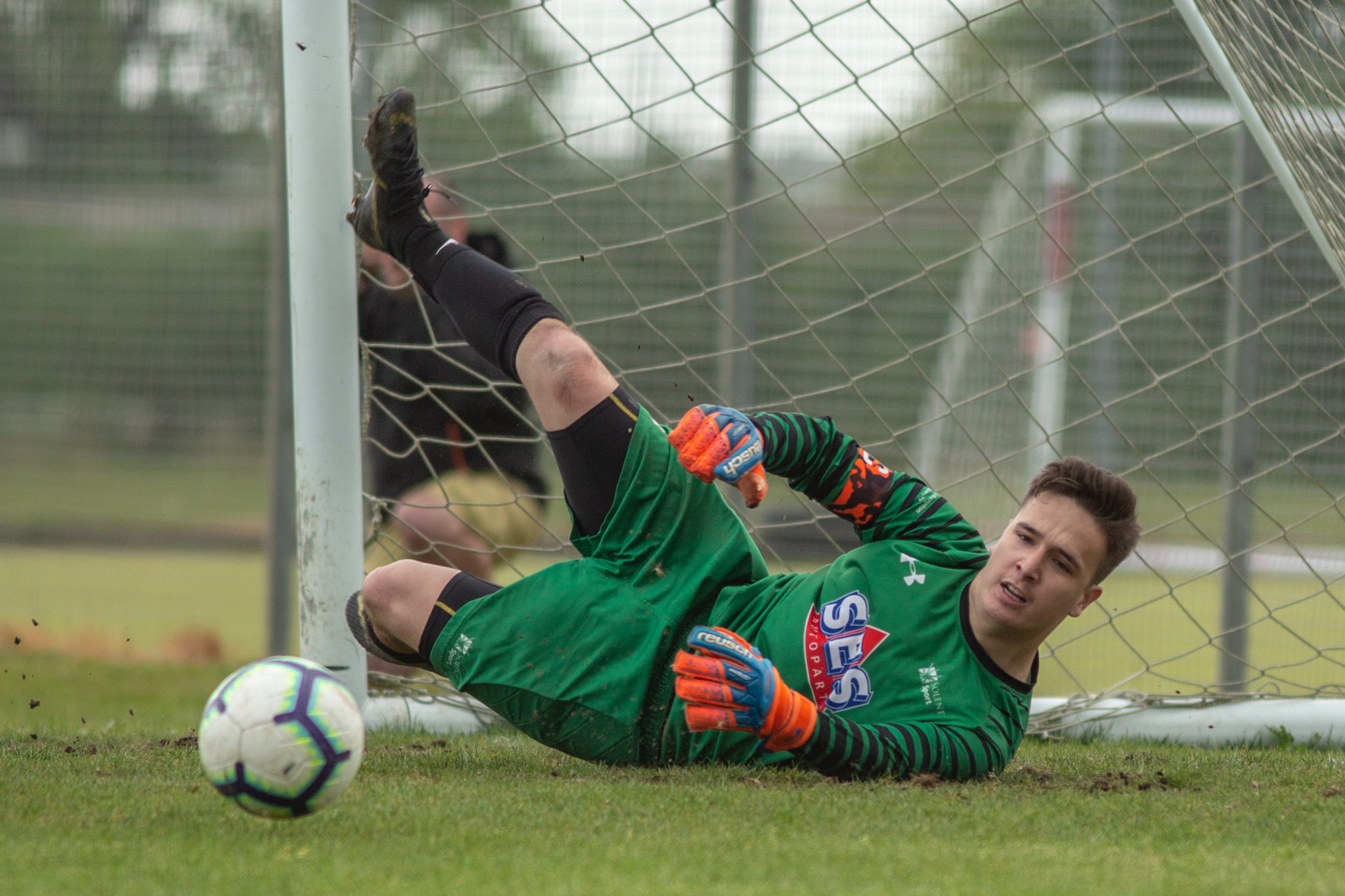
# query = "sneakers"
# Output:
<box><xmin>346</xmin><ymin>86</ymin><xmax>450</xmax><ymax>260</ymax></box>
<box><xmin>343</xmin><ymin>591</ymin><xmax>445</xmax><ymax>676</ymax></box>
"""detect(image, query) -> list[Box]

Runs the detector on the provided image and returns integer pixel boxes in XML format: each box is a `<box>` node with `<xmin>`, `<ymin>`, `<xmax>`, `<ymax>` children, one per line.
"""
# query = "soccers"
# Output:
<box><xmin>199</xmin><ymin>654</ymin><xmax>363</xmax><ymax>820</ymax></box>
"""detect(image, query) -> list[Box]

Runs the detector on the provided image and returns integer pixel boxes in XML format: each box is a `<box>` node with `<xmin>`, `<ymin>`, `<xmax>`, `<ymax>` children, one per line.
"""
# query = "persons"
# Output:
<box><xmin>357</xmin><ymin>159</ymin><xmax>554</xmax><ymax>689</ymax></box>
<box><xmin>344</xmin><ymin>87</ymin><xmax>1142</xmax><ymax>782</ymax></box>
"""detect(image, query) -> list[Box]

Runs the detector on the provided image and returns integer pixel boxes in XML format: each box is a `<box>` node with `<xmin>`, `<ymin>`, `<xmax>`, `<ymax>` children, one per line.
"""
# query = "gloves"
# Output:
<box><xmin>671</xmin><ymin>623</ymin><xmax>816</xmax><ymax>750</ymax></box>
<box><xmin>668</xmin><ymin>403</ymin><xmax>768</xmax><ymax>508</ymax></box>
